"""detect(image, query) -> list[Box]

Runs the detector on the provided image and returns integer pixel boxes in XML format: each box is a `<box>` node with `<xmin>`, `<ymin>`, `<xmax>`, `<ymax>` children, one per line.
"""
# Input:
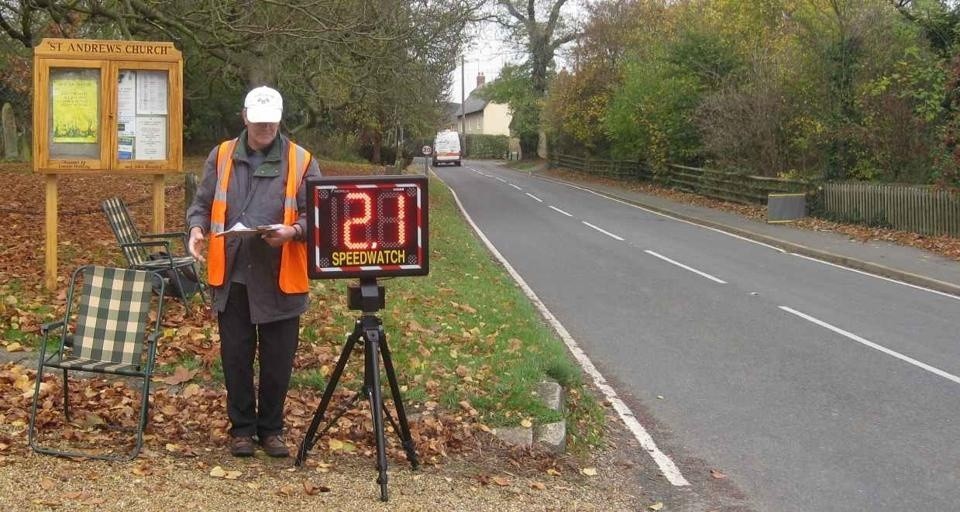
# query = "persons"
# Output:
<box><xmin>182</xmin><ymin>85</ymin><xmax>323</xmax><ymax>458</ymax></box>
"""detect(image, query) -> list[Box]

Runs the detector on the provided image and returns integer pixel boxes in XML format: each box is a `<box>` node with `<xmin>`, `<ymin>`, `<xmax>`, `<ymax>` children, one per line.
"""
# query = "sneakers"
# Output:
<box><xmin>229</xmin><ymin>434</ymin><xmax>291</xmax><ymax>458</ymax></box>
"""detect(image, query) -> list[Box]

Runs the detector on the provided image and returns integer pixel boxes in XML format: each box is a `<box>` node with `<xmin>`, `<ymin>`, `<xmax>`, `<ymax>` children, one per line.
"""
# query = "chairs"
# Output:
<box><xmin>28</xmin><ymin>264</ymin><xmax>166</xmax><ymax>462</ymax></box>
<box><xmin>101</xmin><ymin>194</ymin><xmax>210</xmax><ymax>317</ymax></box>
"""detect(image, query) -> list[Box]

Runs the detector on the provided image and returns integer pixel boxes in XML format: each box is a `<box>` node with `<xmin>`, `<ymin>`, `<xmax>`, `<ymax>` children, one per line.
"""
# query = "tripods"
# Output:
<box><xmin>295</xmin><ymin>316</ymin><xmax>418</xmax><ymax>501</ymax></box>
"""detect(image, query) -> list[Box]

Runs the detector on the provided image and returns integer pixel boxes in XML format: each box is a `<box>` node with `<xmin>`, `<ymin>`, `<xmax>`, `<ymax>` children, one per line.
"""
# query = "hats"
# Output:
<box><xmin>244</xmin><ymin>85</ymin><xmax>284</xmax><ymax>124</ymax></box>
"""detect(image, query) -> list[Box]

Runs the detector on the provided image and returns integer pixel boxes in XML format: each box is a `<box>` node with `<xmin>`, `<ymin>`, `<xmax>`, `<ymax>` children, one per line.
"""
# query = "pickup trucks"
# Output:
<box><xmin>431</xmin><ymin>136</ymin><xmax>462</xmax><ymax>167</ymax></box>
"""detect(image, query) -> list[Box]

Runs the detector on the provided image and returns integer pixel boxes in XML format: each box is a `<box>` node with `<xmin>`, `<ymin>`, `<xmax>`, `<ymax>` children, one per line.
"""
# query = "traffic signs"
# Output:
<box><xmin>421</xmin><ymin>145</ymin><xmax>432</xmax><ymax>155</ymax></box>
